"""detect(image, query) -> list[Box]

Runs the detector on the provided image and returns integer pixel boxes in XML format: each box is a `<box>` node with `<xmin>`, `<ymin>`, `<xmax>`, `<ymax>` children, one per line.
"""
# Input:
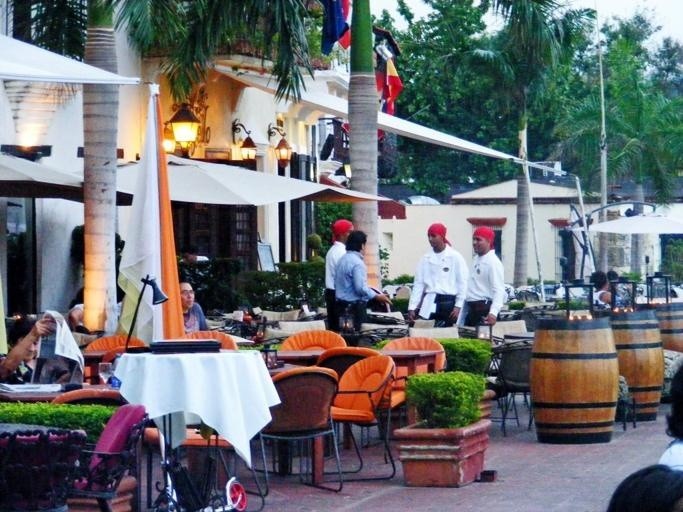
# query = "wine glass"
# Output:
<box><xmin>99</xmin><ymin>362</ymin><xmax>112</xmax><ymax>387</ymax></box>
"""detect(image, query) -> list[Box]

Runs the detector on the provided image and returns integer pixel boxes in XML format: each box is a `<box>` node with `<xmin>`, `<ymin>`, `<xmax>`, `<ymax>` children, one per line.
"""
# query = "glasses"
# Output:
<box><xmin>179</xmin><ymin>289</ymin><xmax>196</xmax><ymax>298</ymax></box>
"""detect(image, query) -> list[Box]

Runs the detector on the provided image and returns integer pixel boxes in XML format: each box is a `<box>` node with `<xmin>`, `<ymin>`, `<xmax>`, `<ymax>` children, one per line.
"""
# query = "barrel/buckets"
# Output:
<box><xmin>658</xmin><ymin>302</ymin><xmax>683</xmax><ymax>355</ymax></box>
<box><xmin>528</xmin><ymin>315</ymin><xmax>620</xmax><ymax>444</ymax></box>
<box><xmin>610</xmin><ymin>307</ymin><xmax>663</xmax><ymax>422</ymax></box>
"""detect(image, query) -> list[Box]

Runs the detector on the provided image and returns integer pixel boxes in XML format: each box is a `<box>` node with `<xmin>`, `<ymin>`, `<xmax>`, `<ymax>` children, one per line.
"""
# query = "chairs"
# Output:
<box><xmin>616</xmin><ymin>371</ymin><xmax>637</xmax><ymax>431</ymax></box>
<box><xmin>380</xmin><ymin>335</ymin><xmax>446</xmax><ymax>466</ymax></box>
<box><xmin>274</xmin><ymin>329</ymin><xmax>347</xmax><ymax>357</ymax></box>
<box><xmin>183</xmin><ymin>328</ymin><xmax>238</xmax><ymax>350</ymax></box>
<box><xmin>70</xmin><ymin>404</ymin><xmax>149</xmax><ymax>512</ymax></box>
<box><xmin>485</xmin><ymin>337</ymin><xmax>541</xmax><ymax>437</ymax></box>
<box><xmin>257</xmin><ymin>309</ymin><xmax>301</xmax><ymax>324</ymax></box>
<box><xmin>298</xmin><ymin>353</ymin><xmax>396</xmax><ymax>484</ymax></box>
<box><xmin>232</xmin><ymin>365</ymin><xmax>344</xmax><ymax>497</ymax></box>
<box><xmin>278</xmin><ymin>319</ymin><xmax>328</xmax><ymax>334</ymax></box>
<box><xmin>316</xmin><ymin>347</ymin><xmax>381</xmax><ymax>376</ymax></box>
<box><xmin>79</xmin><ymin>335</ymin><xmax>149</xmax><ymax>386</ymax></box>
<box><xmin>0</xmin><ymin>428</ymin><xmax>91</xmax><ymax>512</ymax></box>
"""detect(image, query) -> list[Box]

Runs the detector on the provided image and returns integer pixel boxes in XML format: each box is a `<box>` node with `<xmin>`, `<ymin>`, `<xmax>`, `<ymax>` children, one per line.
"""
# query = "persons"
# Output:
<box><xmin>0</xmin><ymin>317</ymin><xmax>72</xmax><ymax>384</ymax></box>
<box><xmin>607</xmin><ymin>271</ymin><xmax>622</xmax><ymax>297</ymax></box>
<box><xmin>464</xmin><ymin>226</ymin><xmax>506</xmax><ymax>327</ymax></box>
<box><xmin>334</xmin><ymin>231</ymin><xmax>392</xmax><ymax>357</ymax></box>
<box><xmin>590</xmin><ymin>272</ymin><xmax>623</xmax><ymax>308</ymax></box>
<box><xmin>179</xmin><ymin>280</ymin><xmax>210</xmax><ymax>334</ymax></box>
<box><xmin>606</xmin><ymin>359</ymin><xmax>683</xmax><ymax>512</ymax></box>
<box><xmin>68</xmin><ymin>304</ymin><xmax>84</xmax><ymax>330</ymax></box>
<box><xmin>324</xmin><ymin>219</ymin><xmax>354</xmax><ymax>332</ymax></box>
<box><xmin>408</xmin><ymin>223</ymin><xmax>468</xmax><ymax>328</ymax></box>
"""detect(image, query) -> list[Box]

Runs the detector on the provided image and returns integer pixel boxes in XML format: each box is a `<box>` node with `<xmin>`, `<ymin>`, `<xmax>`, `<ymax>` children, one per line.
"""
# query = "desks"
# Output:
<box><xmin>274</xmin><ymin>348</ymin><xmax>443</xmax><ymax>424</ymax></box>
<box><xmin>113</xmin><ymin>350</ymin><xmax>282</xmax><ymax>507</ymax></box>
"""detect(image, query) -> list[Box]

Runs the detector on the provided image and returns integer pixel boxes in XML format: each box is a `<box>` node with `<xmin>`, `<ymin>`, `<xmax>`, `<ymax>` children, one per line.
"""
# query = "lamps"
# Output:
<box><xmin>124</xmin><ymin>273</ymin><xmax>169</xmax><ymax>354</ymax></box>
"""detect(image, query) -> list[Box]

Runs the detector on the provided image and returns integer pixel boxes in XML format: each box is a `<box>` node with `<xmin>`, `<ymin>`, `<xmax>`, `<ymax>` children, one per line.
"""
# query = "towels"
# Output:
<box><xmin>418</xmin><ymin>291</ymin><xmax>437</xmax><ymax>321</ymax></box>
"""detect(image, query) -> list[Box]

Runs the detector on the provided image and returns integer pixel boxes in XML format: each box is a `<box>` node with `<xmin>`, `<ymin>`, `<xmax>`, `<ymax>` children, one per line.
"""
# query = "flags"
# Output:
<box><xmin>341</xmin><ymin>66</ymin><xmax>385</xmax><ymax>142</ymax></box>
<box><xmin>339</xmin><ymin>1</ymin><xmax>351</xmax><ymax>50</ymax></box>
<box><xmin>321</xmin><ymin>0</ymin><xmax>349</xmax><ymax>56</ymax></box>
<box><xmin>385</xmin><ymin>58</ymin><xmax>403</xmax><ymax>116</ymax></box>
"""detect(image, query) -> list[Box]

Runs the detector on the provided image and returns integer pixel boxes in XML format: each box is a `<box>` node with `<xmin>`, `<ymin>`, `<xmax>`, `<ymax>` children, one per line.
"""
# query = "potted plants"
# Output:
<box><xmin>392</xmin><ymin>370</ymin><xmax>492</xmax><ymax>488</ymax></box>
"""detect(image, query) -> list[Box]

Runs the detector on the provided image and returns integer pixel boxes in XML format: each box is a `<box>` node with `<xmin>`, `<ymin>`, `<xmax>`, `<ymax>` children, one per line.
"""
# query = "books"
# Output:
<box><xmin>456</xmin><ymin>300</ymin><xmax>469</xmax><ymax>328</ymax></box>
<box><xmin>150</xmin><ymin>339</ymin><xmax>222</xmax><ymax>354</ymax></box>
